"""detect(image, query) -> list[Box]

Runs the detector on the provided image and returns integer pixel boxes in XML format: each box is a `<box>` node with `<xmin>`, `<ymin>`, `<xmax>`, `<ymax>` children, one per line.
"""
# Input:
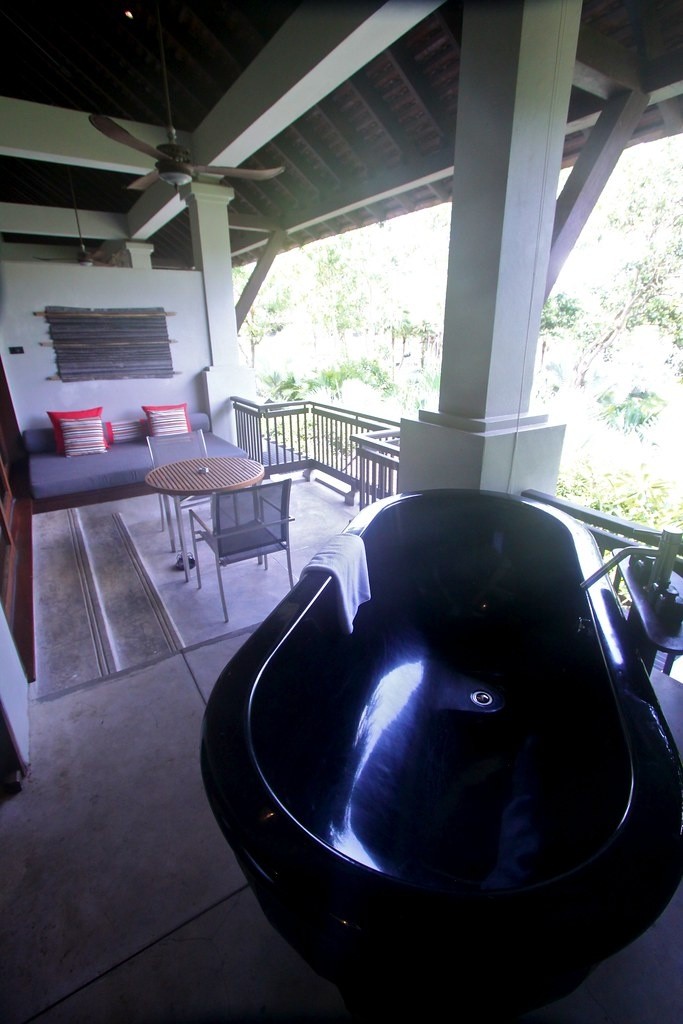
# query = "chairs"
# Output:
<box><xmin>147</xmin><ymin>427</ymin><xmax>218</xmax><ymax>552</ymax></box>
<box><xmin>190</xmin><ymin>476</ymin><xmax>293</xmax><ymax>621</ymax></box>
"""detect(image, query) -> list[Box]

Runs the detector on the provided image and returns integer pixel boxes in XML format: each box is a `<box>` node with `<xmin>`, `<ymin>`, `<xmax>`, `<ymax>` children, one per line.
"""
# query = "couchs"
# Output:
<box><xmin>23</xmin><ymin>412</ymin><xmax>247</xmax><ymax>514</ymax></box>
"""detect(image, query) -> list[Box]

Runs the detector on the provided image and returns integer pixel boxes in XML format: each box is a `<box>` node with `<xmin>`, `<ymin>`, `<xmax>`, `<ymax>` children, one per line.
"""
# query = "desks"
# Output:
<box><xmin>613</xmin><ymin>546</ymin><xmax>683</xmax><ymax>677</ymax></box>
<box><xmin>146</xmin><ymin>458</ymin><xmax>265</xmax><ymax>582</ymax></box>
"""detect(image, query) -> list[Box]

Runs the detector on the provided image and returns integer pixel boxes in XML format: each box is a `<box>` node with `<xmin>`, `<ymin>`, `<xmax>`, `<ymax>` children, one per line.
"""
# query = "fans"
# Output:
<box><xmin>88</xmin><ymin>41</ymin><xmax>285</xmax><ymax>193</ymax></box>
<box><xmin>31</xmin><ymin>165</ymin><xmax>114</xmax><ymax>269</ymax></box>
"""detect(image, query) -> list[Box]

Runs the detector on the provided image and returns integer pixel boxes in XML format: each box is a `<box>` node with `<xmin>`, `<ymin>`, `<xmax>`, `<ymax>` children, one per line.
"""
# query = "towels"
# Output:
<box><xmin>298</xmin><ymin>532</ymin><xmax>373</xmax><ymax>636</ymax></box>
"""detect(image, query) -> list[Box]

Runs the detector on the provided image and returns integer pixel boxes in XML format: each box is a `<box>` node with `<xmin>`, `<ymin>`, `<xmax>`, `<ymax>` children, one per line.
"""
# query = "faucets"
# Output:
<box><xmin>579</xmin><ymin>526</ymin><xmax>683</xmax><ymax>604</ymax></box>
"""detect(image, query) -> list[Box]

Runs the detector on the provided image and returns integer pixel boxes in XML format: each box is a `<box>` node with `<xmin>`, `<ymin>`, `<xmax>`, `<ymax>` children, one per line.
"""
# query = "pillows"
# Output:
<box><xmin>47</xmin><ymin>406</ymin><xmax>110</xmax><ymax>455</ymax></box>
<box><xmin>110</xmin><ymin>418</ymin><xmax>146</xmax><ymax>443</ymax></box>
<box><xmin>58</xmin><ymin>417</ymin><xmax>108</xmax><ymax>458</ymax></box>
<box><xmin>141</xmin><ymin>403</ymin><xmax>191</xmax><ymax>437</ymax></box>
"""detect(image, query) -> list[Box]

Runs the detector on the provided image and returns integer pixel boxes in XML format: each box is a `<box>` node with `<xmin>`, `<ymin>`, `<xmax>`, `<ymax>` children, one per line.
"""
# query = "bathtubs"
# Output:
<box><xmin>198</xmin><ymin>488</ymin><xmax>683</xmax><ymax>1024</ymax></box>
